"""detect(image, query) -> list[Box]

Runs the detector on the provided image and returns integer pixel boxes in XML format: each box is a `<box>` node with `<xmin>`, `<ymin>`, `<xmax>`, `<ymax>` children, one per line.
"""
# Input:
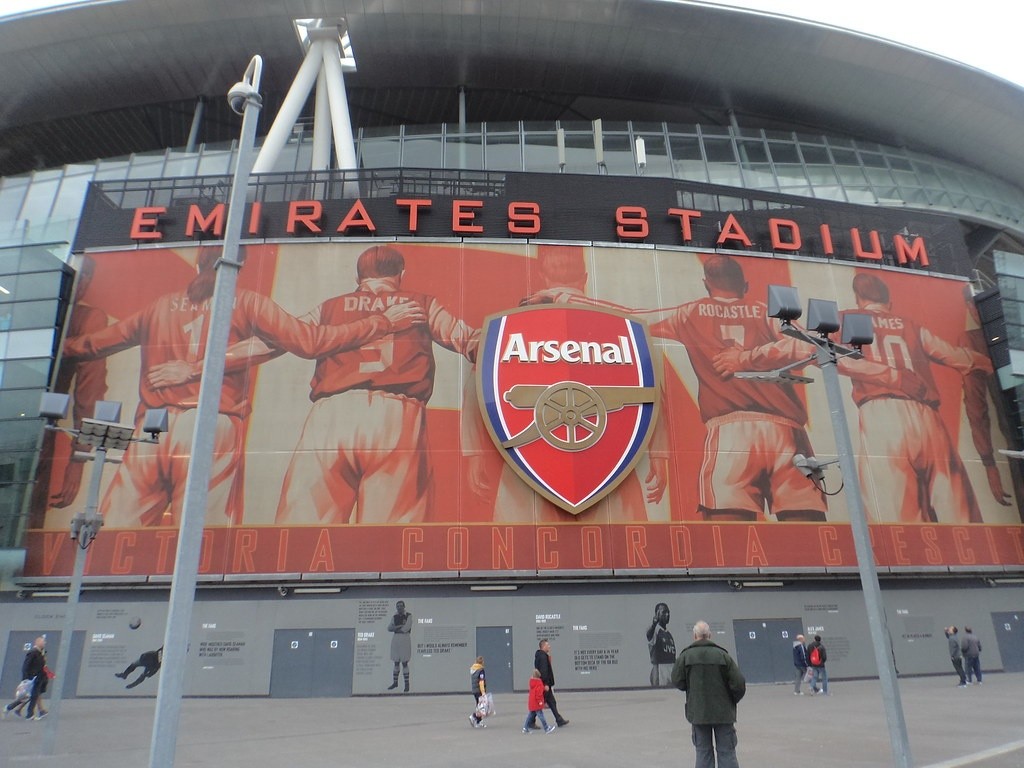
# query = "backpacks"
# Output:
<box><xmin>810</xmin><ymin>644</ymin><xmax>823</xmax><ymax>665</ymax></box>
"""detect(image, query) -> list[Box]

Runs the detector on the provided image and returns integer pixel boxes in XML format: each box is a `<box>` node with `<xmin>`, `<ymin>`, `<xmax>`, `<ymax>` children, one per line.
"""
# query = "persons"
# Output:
<box><xmin>670</xmin><ymin>621</ymin><xmax>746</xmax><ymax>768</ymax></box>
<box><xmin>941</xmin><ymin>628</ymin><xmax>986</xmax><ymax>688</ymax></box>
<box><xmin>645</xmin><ymin>603</ymin><xmax>677</xmax><ymax>688</ymax></box>
<box><xmin>386</xmin><ymin>601</ymin><xmax>412</xmax><ymax>692</ymax></box>
<box><xmin>461</xmin><ymin>635</ymin><xmax>570</xmax><ymax>733</ymax></box>
<box><xmin>789</xmin><ymin>632</ymin><xmax>826</xmax><ymax>690</ymax></box>
<box><xmin>2</xmin><ymin>637</ymin><xmax>49</xmax><ymax>722</ymax></box>
<box><xmin>35</xmin><ymin>245</ymin><xmax>1011</xmax><ymax>528</ymax></box>
<box><xmin>115</xmin><ymin>646</ymin><xmax>163</xmax><ymax>690</ymax></box>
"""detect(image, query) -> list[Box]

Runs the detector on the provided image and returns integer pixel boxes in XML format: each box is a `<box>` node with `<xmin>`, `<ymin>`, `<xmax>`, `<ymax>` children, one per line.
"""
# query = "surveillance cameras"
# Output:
<box><xmin>228</xmin><ymin>81</ymin><xmax>252</xmax><ymax>114</ymax></box>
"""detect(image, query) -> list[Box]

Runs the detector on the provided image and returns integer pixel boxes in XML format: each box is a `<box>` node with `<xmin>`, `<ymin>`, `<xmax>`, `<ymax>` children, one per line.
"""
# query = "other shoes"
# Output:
<box><xmin>3</xmin><ymin>705</ymin><xmax>9</xmax><ymax>718</ymax></box>
<box><xmin>816</xmin><ymin>689</ymin><xmax>823</xmax><ymax>694</ymax></box>
<box><xmin>478</xmin><ymin>720</ymin><xmax>487</xmax><ymax>728</ymax></box>
<box><xmin>810</xmin><ymin>687</ymin><xmax>814</xmax><ymax>696</ymax></box>
<box><xmin>794</xmin><ymin>692</ymin><xmax>804</xmax><ymax>696</ymax></box>
<box><xmin>26</xmin><ymin>715</ymin><xmax>39</xmax><ymax>721</ymax></box>
<box><xmin>37</xmin><ymin>710</ymin><xmax>48</xmax><ymax>720</ymax></box>
<box><xmin>546</xmin><ymin>725</ymin><xmax>556</xmax><ymax>734</ymax></box>
<box><xmin>958</xmin><ymin>684</ymin><xmax>966</xmax><ymax>688</ymax></box>
<box><xmin>967</xmin><ymin>681</ymin><xmax>973</xmax><ymax>685</ymax></box>
<box><xmin>558</xmin><ymin>719</ymin><xmax>569</xmax><ymax>727</ymax></box>
<box><xmin>529</xmin><ymin>724</ymin><xmax>540</xmax><ymax>729</ymax></box>
<box><xmin>522</xmin><ymin>728</ymin><xmax>533</xmax><ymax>734</ymax></box>
<box><xmin>13</xmin><ymin>710</ymin><xmax>22</xmax><ymax>718</ymax></box>
<box><xmin>978</xmin><ymin>681</ymin><xmax>982</xmax><ymax>685</ymax></box>
<box><xmin>469</xmin><ymin>714</ymin><xmax>476</xmax><ymax>727</ymax></box>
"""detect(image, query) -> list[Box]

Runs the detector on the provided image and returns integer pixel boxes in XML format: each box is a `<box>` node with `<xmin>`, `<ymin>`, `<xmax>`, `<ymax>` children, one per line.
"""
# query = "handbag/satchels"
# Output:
<box><xmin>16</xmin><ymin>676</ymin><xmax>37</xmax><ymax>703</ymax></box>
<box><xmin>476</xmin><ymin>692</ymin><xmax>496</xmax><ymax>719</ymax></box>
<box><xmin>804</xmin><ymin>667</ymin><xmax>816</xmax><ymax>682</ymax></box>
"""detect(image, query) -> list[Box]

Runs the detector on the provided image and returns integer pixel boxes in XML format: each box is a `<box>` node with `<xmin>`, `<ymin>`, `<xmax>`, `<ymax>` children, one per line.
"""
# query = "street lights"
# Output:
<box><xmin>36</xmin><ymin>392</ymin><xmax>171</xmax><ymax>699</ymax></box>
<box><xmin>765</xmin><ymin>282</ymin><xmax>916</xmax><ymax>768</ymax></box>
<box><xmin>147</xmin><ymin>49</ymin><xmax>266</xmax><ymax>768</ymax></box>
